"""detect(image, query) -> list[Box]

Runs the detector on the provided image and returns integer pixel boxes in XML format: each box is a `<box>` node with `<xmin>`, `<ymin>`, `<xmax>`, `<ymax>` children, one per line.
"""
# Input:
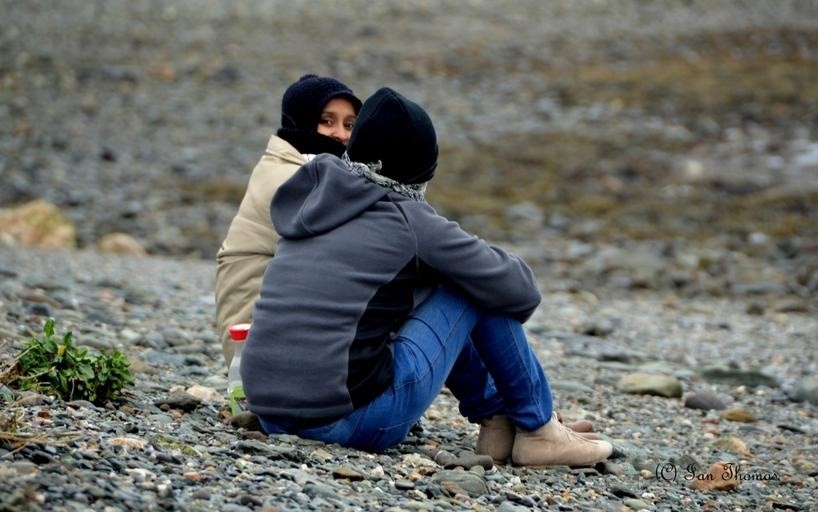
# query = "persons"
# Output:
<box><xmin>212</xmin><ymin>72</ymin><xmax>363</xmax><ymax>370</ymax></box>
<box><xmin>238</xmin><ymin>87</ymin><xmax>615</xmax><ymax>468</ymax></box>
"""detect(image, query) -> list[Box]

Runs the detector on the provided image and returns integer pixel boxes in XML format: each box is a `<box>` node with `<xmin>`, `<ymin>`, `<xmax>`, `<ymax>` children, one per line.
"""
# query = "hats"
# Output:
<box><xmin>281</xmin><ymin>74</ymin><xmax>439</xmax><ymax>184</ymax></box>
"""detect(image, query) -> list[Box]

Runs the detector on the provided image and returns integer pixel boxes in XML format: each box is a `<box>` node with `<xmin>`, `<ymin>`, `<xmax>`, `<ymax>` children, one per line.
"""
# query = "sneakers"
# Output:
<box><xmin>476</xmin><ymin>410</ymin><xmax>613</xmax><ymax>464</ymax></box>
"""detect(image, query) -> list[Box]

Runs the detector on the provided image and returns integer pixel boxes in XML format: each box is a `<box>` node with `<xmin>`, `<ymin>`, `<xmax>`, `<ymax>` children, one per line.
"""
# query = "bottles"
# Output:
<box><xmin>228</xmin><ymin>324</ymin><xmax>250</xmax><ymax>418</ymax></box>
